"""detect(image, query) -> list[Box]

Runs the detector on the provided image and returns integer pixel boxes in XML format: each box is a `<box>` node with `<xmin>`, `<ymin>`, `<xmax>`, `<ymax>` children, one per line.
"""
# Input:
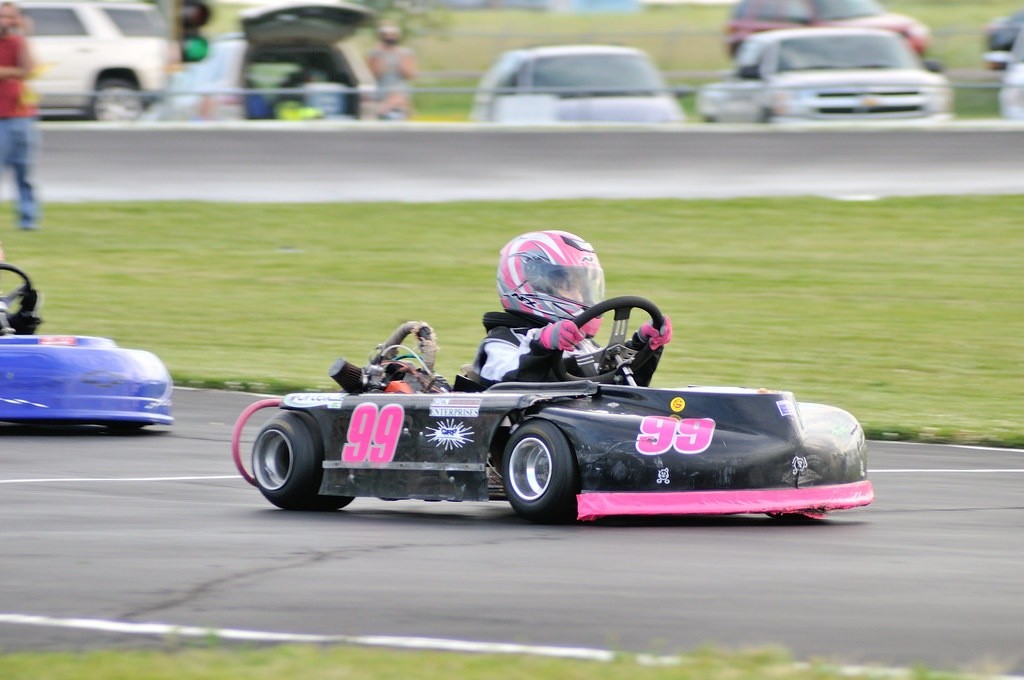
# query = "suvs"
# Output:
<box><xmin>10</xmin><ymin>0</ymin><xmax>182</xmax><ymax>122</ymax></box>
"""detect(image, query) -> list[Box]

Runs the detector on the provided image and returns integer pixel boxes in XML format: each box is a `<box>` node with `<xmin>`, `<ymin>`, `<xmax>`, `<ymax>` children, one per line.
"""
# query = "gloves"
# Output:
<box><xmin>539</xmin><ymin>319</ymin><xmax>584</xmax><ymax>352</ymax></box>
<box><xmin>636</xmin><ymin>315</ymin><xmax>673</xmax><ymax>350</ymax></box>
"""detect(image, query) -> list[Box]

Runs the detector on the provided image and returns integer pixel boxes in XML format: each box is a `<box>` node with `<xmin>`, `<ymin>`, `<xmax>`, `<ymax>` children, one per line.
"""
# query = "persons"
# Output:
<box><xmin>364</xmin><ymin>25</ymin><xmax>418</xmax><ymax>121</ymax></box>
<box><xmin>0</xmin><ymin>284</ymin><xmax>42</xmax><ymax>334</ymax></box>
<box><xmin>0</xmin><ymin>2</ymin><xmax>41</xmax><ymax>231</ymax></box>
<box><xmin>272</xmin><ymin>72</ymin><xmax>327</xmax><ymax>121</ymax></box>
<box><xmin>475</xmin><ymin>227</ymin><xmax>673</xmax><ymax>390</ymax></box>
<box><xmin>174</xmin><ymin>6</ymin><xmax>209</xmax><ymax>64</ymax></box>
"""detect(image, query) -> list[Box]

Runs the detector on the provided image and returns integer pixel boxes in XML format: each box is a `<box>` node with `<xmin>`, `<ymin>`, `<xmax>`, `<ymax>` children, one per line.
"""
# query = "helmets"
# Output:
<box><xmin>494</xmin><ymin>230</ymin><xmax>606</xmax><ymax>335</ymax></box>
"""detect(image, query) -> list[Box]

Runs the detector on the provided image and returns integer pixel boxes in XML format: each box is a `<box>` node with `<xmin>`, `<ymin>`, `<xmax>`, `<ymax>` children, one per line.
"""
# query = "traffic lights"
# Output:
<box><xmin>169</xmin><ymin>0</ymin><xmax>213</xmax><ymax>67</ymax></box>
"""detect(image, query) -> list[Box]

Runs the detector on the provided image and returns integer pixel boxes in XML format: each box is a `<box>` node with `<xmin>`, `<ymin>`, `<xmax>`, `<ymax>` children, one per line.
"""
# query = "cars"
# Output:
<box><xmin>985</xmin><ymin>18</ymin><xmax>1024</xmax><ymax>120</ymax></box>
<box><xmin>691</xmin><ymin>25</ymin><xmax>956</xmax><ymax>124</ymax></box>
<box><xmin>468</xmin><ymin>42</ymin><xmax>689</xmax><ymax>124</ymax></box>
<box><xmin>723</xmin><ymin>0</ymin><xmax>933</xmax><ymax>58</ymax></box>
<box><xmin>160</xmin><ymin>1</ymin><xmax>381</xmax><ymax>123</ymax></box>
